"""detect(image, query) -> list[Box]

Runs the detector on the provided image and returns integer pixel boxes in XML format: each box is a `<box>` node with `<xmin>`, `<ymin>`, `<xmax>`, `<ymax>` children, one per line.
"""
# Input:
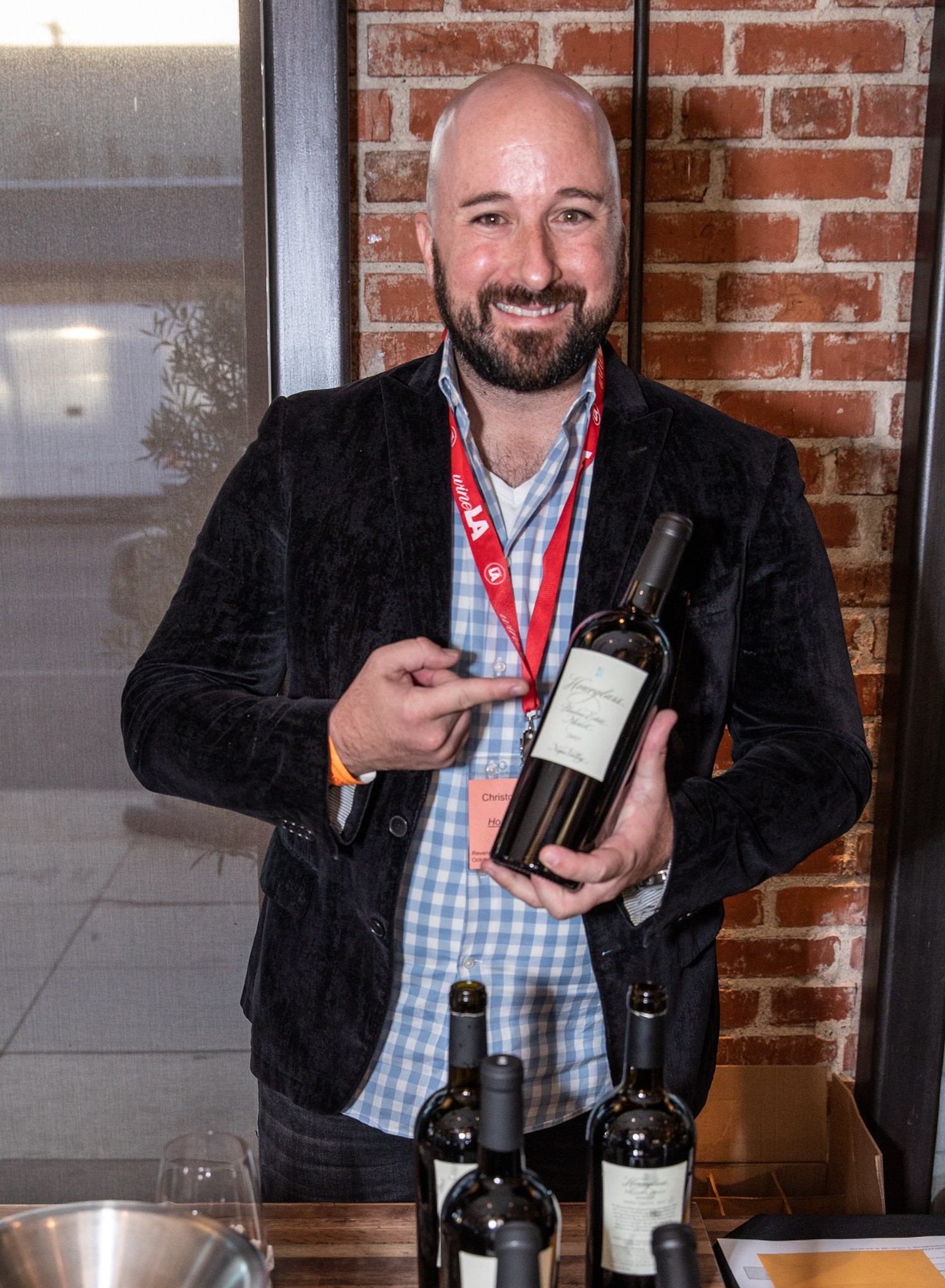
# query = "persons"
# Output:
<box><xmin>122</xmin><ymin>62</ymin><xmax>872</xmax><ymax>1206</ymax></box>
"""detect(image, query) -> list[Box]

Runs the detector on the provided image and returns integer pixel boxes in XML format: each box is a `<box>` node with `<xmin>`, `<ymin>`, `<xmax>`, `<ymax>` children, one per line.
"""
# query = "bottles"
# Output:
<box><xmin>414</xmin><ymin>975</ymin><xmax>702</xmax><ymax>1288</ymax></box>
<box><xmin>490</xmin><ymin>509</ymin><xmax>702</xmax><ymax>895</ymax></box>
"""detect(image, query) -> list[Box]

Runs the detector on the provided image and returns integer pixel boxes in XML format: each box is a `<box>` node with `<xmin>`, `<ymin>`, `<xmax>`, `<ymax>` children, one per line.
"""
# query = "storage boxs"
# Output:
<box><xmin>693</xmin><ymin>1064</ymin><xmax>886</xmax><ymax>1246</ymax></box>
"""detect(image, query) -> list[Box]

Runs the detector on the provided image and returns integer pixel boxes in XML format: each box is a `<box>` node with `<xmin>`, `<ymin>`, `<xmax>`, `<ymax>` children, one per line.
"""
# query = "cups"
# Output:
<box><xmin>156</xmin><ymin>1133</ymin><xmax>268</xmax><ymax>1265</ymax></box>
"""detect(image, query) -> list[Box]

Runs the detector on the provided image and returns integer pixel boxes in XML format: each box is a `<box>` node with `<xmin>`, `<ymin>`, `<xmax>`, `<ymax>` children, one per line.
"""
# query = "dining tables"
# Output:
<box><xmin>0</xmin><ymin>1202</ymin><xmax>726</xmax><ymax>1288</ymax></box>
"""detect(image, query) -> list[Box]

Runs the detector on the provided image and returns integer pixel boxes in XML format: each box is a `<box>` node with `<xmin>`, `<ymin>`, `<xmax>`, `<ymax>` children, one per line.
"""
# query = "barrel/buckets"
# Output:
<box><xmin>0</xmin><ymin>1200</ymin><xmax>265</xmax><ymax>1287</ymax></box>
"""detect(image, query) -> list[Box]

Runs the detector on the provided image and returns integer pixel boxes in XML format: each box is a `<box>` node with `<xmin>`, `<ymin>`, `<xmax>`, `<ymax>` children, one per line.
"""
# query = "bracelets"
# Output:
<box><xmin>639</xmin><ymin>867</ymin><xmax>667</xmax><ymax>889</ymax></box>
